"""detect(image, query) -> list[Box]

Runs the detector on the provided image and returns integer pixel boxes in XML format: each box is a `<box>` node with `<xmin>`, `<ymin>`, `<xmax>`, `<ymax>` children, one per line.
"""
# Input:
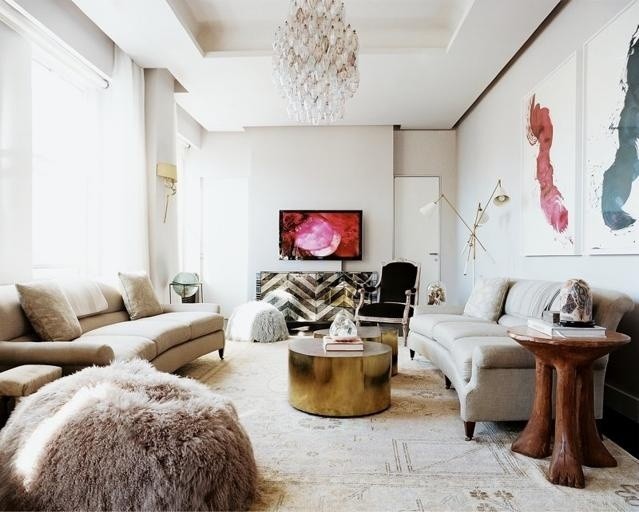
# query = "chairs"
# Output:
<box><xmin>352</xmin><ymin>257</ymin><xmax>422</xmax><ymax>345</ymax></box>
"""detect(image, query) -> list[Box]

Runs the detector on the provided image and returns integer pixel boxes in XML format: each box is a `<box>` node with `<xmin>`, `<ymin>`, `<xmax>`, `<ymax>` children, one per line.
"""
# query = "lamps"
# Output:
<box><xmin>156</xmin><ymin>162</ymin><xmax>178</xmax><ymax>224</ymax></box>
<box><xmin>269</xmin><ymin>0</ymin><xmax>361</xmax><ymax>126</ymax></box>
<box><xmin>420</xmin><ymin>178</ymin><xmax>511</xmax><ymax>290</ymax></box>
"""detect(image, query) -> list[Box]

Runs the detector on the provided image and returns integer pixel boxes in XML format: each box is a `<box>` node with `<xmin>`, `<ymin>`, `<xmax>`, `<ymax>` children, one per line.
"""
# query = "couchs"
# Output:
<box><xmin>0</xmin><ymin>279</ymin><xmax>226</xmax><ymax>376</ymax></box>
<box><xmin>406</xmin><ymin>278</ymin><xmax>634</xmax><ymax>442</ymax></box>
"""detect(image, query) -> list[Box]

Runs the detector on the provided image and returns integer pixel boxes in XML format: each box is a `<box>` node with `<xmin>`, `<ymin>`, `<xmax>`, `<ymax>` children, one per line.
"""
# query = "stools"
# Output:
<box><xmin>0</xmin><ymin>364</ymin><xmax>62</xmax><ymax>408</ymax></box>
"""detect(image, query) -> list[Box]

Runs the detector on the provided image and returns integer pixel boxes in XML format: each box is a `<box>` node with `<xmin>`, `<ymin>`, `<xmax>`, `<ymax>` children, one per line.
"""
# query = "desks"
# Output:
<box><xmin>504</xmin><ymin>327</ymin><xmax>632</xmax><ymax>490</ymax></box>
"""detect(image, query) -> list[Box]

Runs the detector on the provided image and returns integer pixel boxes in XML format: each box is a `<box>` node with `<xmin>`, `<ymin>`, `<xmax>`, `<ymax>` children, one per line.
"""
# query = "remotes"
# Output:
<box><xmin>560</xmin><ymin>320</ymin><xmax>595</xmax><ymax>327</ymax></box>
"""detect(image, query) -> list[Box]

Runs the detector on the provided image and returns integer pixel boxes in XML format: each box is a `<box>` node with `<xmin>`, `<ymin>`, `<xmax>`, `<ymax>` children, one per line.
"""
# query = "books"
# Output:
<box><xmin>527</xmin><ymin>317</ymin><xmax>607</xmax><ymax>340</ymax></box>
<box><xmin>322</xmin><ymin>335</ymin><xmax>363</xmax><ymax>351</ymax></box>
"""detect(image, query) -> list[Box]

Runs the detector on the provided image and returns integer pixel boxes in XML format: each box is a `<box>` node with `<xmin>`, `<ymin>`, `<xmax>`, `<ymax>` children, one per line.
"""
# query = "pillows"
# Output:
<box><xmin>11</xmin><ymin>280</ymin><xmax>94</xmax><ymax>343</ymax></box>
<box><xmin>118</xmin><ymin>268</ymin><xmax>164</xmax><ymax>321</ymax></box>
<box><xmin>463</xmin><ymin>278</ymin><xmax>510</xmax><ymax>322</ymax></box>
